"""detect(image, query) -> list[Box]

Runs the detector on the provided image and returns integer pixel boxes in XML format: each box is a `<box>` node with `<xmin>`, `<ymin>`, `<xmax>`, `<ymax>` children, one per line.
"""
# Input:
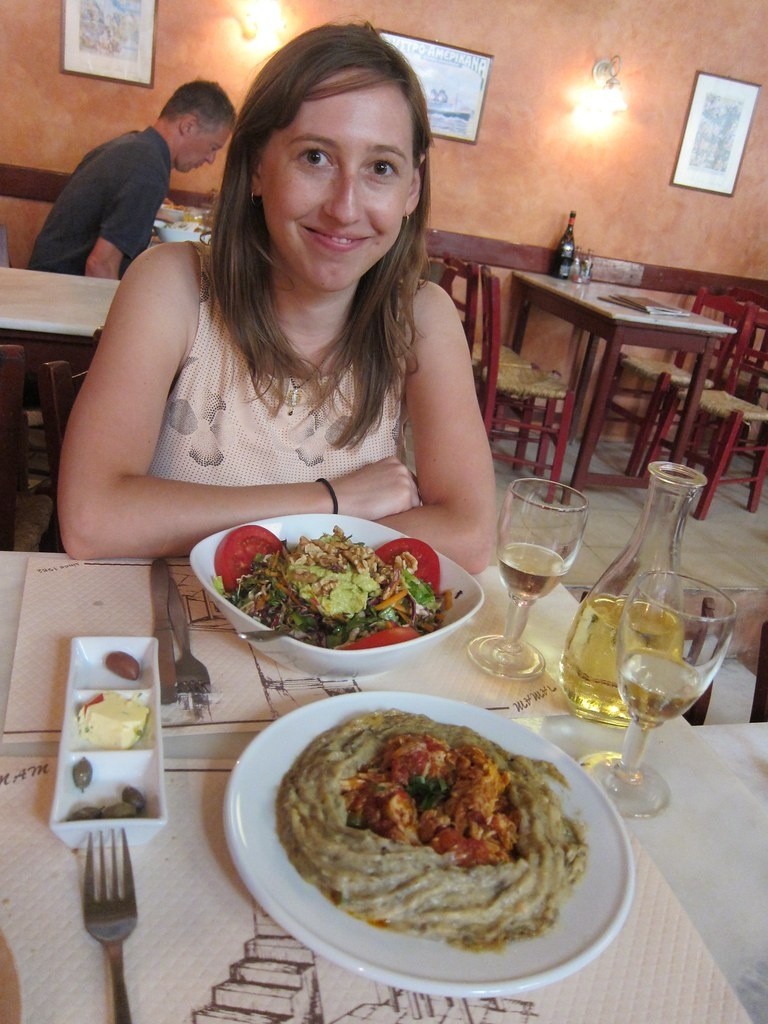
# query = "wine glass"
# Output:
<box><xmin>468</xmin><ymin>477</ymin><xmax>590</xmax><ymax>682</ymax></box>
<box><xmin>574</xmin><ymin>571</ymin><xmax>737</xmax><ymax>818</ymax></box>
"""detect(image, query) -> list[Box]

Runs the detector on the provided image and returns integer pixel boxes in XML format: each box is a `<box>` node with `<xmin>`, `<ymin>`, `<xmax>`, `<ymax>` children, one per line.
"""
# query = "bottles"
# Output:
<box><xmin>552</xmin><ymin>210</ymin><xmax>576</xmax><ymax>279</ymax></box>
<box><xmin>558</xmin><ymin>461</ymin><xmax>708</xmax><ymax>729</ymax></box>
<box><xmin>569</xmin><ymin>246</ymin><xmax>594</xmax><ymax>284</ymax></box>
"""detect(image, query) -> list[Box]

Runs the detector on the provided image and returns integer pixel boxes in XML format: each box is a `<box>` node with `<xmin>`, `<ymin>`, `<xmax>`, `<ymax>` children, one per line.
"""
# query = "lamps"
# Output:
<box><xmin>591</xmin><ymin>54</ymin><xmax>626</xmax><ymax>113</ymax></box>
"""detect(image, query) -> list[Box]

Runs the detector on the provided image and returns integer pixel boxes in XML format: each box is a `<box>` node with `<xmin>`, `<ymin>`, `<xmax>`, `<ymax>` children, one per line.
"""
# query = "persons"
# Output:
<box><xmin>28</xmin><ymin>81</ymin><xmax>236</xmax><ymax>278</ymax></box>
<box><xmin>58</xmin><ymin>23</ymin><xmax>500</xmax><ymax>578</ymax></box>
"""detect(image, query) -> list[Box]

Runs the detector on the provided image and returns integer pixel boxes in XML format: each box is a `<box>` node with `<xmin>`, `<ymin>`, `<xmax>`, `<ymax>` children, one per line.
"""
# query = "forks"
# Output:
<box><xmin>83</xmin><ymin>827</ymin><xmax>138</xmax><ymax>1024</ymax></box>
<box><xmin>167</xmin><ymin>577</ymin><xmax>212</xmax><ymax>695</ymax></box>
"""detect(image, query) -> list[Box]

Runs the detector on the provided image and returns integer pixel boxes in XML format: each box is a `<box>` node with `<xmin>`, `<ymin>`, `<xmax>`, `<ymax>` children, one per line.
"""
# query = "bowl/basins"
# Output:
<box><xmin>154</xmin><ymin>219</ymin><xmax>212</xmax><ymax>244</ymax></box>
<box><xmin>159</xmin><ymin>203</ymin><xmax>210</xmax><ymax>221</ymax></box>
<box><xmin>189</xmin><ymin>513</ymin><xmax>485</xmax><ymax>679</ymax></box>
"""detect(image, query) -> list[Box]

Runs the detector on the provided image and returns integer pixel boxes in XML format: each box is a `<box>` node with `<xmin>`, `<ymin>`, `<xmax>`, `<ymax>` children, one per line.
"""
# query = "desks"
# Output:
<box><xmin>0</xmin><ymin>263</ymin><xmax>123</xmax><ymax>372</ymax></box>
<box><xmin>0</xmin><ymin>547</ymin><xmax>767</xmax><ymax>1024</ymax></box>
<box><xmin>501</xmin><ymin>271</ymin><xmax>738</xmax><ymax>505</ymax></box>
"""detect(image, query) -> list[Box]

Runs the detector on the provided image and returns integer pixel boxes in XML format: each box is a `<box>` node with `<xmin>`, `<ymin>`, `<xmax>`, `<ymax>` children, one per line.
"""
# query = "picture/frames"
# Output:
<box><xmin>668</xmin><ymin>72</ymin><xmax>763</xmax><ymax>197</ymax></box>
<box><xmin>372</xmin><ymin>28</ymin><xmax>494</xmax><ymax>146</ymax></box>
<box><xmin>58</xmin><ymin>1</ymin><xmax>159</xmax><ymax>88</ymax></box>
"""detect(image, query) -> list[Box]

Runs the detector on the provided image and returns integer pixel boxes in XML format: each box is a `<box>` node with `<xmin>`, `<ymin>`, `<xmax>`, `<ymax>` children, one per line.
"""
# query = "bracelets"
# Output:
<box><xmin>315</xmin><ymin>478</ymin><xmax>338</xmax><ymax>514</ymax></box>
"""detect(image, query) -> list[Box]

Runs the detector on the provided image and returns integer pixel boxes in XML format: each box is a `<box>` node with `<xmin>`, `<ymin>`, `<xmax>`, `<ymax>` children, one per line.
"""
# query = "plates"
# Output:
<box><xmin>222</xmin><ymin>692</ymin><xmax>635</xmax><ymax>998</ymax></box>
<box><xmin>49</xmin><ymin>634</ymin><xmax>169</xmax><ymax>848</ymax></box>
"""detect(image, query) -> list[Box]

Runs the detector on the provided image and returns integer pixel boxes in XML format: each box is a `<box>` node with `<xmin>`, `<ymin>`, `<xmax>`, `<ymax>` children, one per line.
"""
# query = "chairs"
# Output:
<box><xmin>442</xmin><ymin>251</ymin><xmax>767</xmax><ymax>521</ymax></box>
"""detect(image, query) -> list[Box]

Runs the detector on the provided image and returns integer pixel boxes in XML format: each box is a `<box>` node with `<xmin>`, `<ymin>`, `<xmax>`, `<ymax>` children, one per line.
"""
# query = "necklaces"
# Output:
<box><xmin>289</xmin><ymin>355</ymin><xmax>327</xmax><ymax>416</ymax></box>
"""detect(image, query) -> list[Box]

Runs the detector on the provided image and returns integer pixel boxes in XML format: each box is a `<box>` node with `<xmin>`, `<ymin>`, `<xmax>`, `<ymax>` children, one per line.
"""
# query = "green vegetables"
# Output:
<box><xmin>215</xmin><ymin>551</ymin><xmax>441</xmax><ymax>648</ymax></box>
<box><xmin>403</xmin><ymin>773</ymin><xmax>451</xmax><ymax>813</ymax></box>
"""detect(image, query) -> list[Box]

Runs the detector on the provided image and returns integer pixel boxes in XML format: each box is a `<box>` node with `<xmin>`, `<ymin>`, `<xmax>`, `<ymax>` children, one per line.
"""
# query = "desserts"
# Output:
<box><xmin>79</xmin><ymin>691</ymin><xmax>151</xmax><ymax>751</ymax></box>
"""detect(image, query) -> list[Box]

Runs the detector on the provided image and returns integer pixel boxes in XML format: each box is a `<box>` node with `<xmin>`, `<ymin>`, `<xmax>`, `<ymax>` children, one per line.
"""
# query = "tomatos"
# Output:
<box><xmin>373</xmin><ymin>539</ymin><xmax>440</xmax><ymax>594</ymax></box>
<box><xmin>215</xmin><ymin>524</ymin><xmax>284</xmax><ymax>591</ymax></box>
<box><xmin>340</xmin><ymin>627</ymin><xmax>420</xmax><ymax>650</ymax></box>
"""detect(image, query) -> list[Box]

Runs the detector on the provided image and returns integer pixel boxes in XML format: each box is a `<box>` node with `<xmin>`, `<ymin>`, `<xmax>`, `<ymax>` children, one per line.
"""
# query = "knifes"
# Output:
<box><xmin>150</xmin><ymin>558</ymin><xmax>177</xmax><ymax>704</ymax></box>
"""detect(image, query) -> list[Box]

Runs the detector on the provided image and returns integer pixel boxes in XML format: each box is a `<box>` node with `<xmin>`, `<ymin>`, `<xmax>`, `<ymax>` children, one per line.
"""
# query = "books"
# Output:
<box><xmin>598</xmin><ymin>295</ymin><xmax>691</xmax><ymax>317</ymax></box>
